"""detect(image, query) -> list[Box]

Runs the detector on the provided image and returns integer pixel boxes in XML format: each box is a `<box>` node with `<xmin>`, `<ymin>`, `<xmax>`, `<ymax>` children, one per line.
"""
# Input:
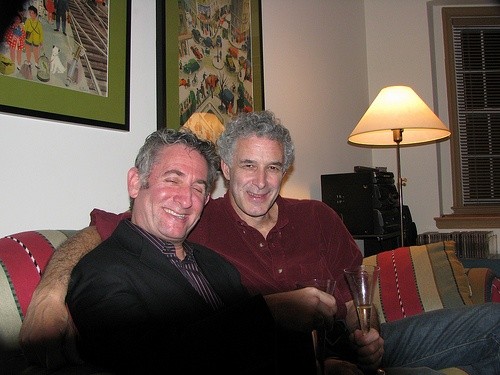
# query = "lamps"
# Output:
<box><xmin>347</xmin><ymin>85</ymin><xmax>450</xmax><ymax>248</ymax></box>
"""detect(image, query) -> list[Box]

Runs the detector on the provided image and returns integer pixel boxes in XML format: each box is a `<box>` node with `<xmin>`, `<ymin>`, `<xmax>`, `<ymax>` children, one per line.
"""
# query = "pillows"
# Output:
<box><xmin>361</xmin><ymin>241</ymin><xmax>476</xmax><ymax>323</ymax></box>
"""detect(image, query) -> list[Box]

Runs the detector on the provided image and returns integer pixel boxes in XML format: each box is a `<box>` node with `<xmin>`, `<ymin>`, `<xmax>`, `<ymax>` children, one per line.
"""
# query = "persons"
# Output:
<box><xmin>18</xmin><ymin>110</ymin><xmax>500</xmax><ymax>375</ymax></box>
<box><xmin>63</xmin><ymin>129</ymin><xmax>469</xmax><ymax>375</ymax></box>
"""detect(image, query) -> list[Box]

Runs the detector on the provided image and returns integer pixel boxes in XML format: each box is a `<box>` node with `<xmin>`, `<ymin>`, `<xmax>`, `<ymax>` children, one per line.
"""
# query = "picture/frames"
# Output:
<box><xmin>155</xmin><ymin>0</ymin><xmax>266</xmax><ymax>133</ymax></box>
<box><xmin>0</xmin><ymin>0</ymin><xmax>132</xmax><ymax>133</ymax></box>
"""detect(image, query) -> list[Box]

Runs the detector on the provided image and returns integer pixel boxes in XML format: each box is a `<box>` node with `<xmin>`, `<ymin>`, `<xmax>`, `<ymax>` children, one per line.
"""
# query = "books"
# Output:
<box><xmin>417</xmin><ymin>231</ymin><xmax>497</xmax><ymax>260</ymax></box>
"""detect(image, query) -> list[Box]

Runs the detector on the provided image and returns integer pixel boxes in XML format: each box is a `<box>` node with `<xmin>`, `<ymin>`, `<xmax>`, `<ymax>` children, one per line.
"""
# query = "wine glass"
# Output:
<box><xmin>296</xmin><ymin>281</ymin><xmax>337</xmax><ymax>375</ymax></box>
<box><xmin>344</xmin><ymin>266</ymin><xmax>381</xmax><ymax>336</ymax></box>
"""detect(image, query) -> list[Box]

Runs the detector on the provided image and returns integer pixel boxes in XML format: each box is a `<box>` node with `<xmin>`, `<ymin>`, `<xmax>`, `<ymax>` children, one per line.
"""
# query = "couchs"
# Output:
<box><xmin>0</xmin><ymin>229</ymin><xmax>500</xmax><ymax>375</ymax></box>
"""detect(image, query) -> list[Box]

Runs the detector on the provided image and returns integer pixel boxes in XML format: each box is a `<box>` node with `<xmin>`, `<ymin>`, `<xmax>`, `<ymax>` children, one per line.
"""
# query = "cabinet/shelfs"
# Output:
<box><xmin>351</xmin><ymin>231</ymin><xmax>401</xmax><ymax>259</ymax></box>
